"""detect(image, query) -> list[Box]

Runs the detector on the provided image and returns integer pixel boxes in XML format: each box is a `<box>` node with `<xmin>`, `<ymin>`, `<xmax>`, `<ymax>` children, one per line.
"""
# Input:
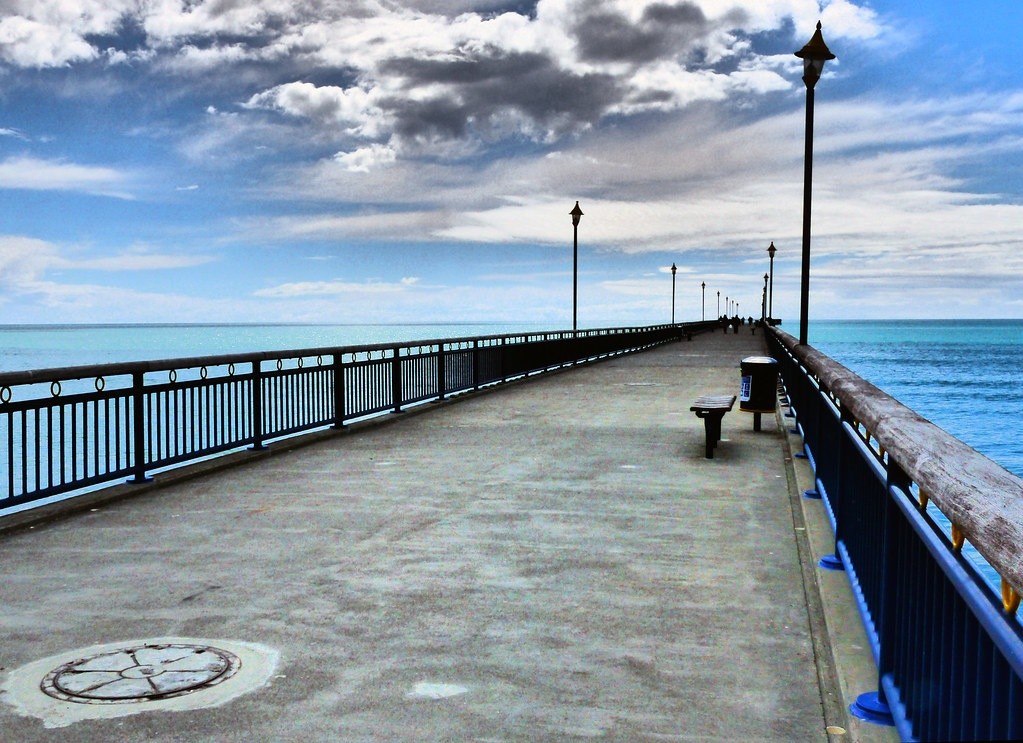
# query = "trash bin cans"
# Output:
<box><xmin>740</xmin><ymin>356</ymin><xmax>780</xmax><ymax>412</ymax></box>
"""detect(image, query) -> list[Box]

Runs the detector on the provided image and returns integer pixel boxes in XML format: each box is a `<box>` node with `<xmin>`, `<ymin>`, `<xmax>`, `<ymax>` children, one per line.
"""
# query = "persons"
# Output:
<box><xmin>748</xmin><ymin>317</ymin><xmax>760</xmax><ymax>335</ymax></box>
<box><xmin>719</xmin><ymin>314</ymin><xmax>745</xmax><ymax>335</ymax></box>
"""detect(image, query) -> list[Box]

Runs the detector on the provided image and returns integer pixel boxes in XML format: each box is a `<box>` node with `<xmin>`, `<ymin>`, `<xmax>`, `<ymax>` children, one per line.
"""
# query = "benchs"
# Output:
<box><xmin>690</xmin><ymin>394</ymin><xmax>736</xmax><ymax>459</ymax></box>
